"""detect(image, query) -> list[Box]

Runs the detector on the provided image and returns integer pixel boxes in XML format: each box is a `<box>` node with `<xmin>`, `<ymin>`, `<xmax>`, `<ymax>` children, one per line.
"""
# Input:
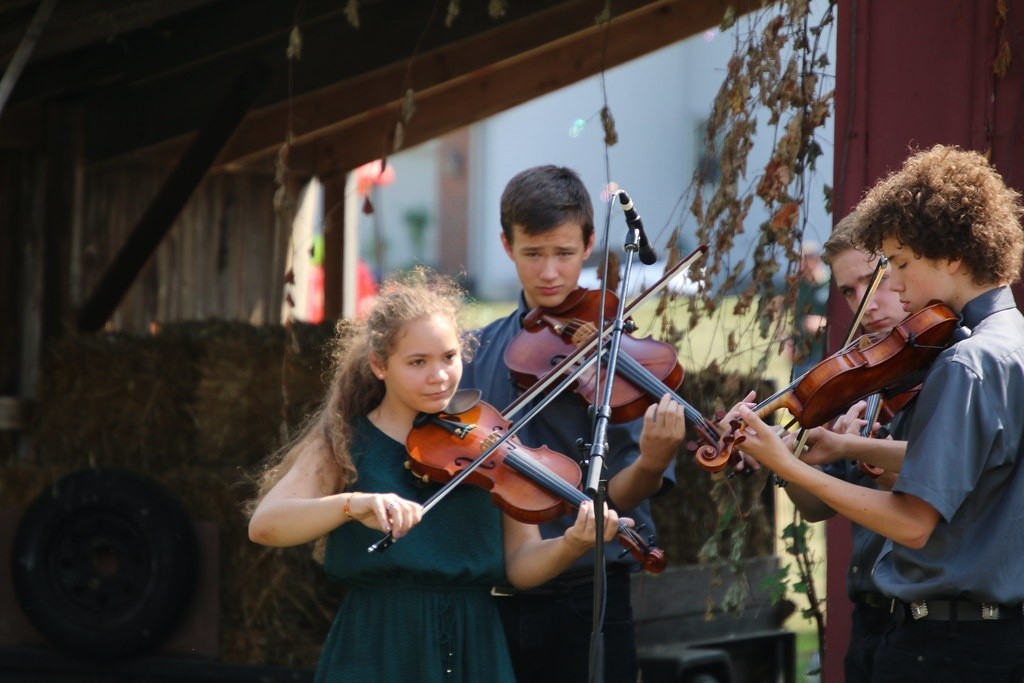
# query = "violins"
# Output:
<box><xmin>403</xmin><ymin>386</ymin><xmax>667</xmax><ymax>574</ymax></box>
<box><xmin>504</xmin><ymin>284</ymin><xmax>760</xmax><ymax>478</ymax></box>
<box><xmin>691</xmin><ymin>300</ymin><xmax>961</xmax><ymax>474</ymax></box>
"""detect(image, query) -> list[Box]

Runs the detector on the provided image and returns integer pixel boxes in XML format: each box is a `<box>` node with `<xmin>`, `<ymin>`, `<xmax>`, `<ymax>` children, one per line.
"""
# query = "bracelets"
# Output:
<box><xmin>343</xmin><ymin>491</ymin><xmax>361</xmax><ymax>521</ymax></box>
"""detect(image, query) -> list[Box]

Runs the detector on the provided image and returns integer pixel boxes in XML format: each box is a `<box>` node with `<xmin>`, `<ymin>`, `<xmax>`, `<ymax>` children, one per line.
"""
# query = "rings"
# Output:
<box><xmin>386</xmin><ymin>503</ymin><xmax>398</xmax><ymax>509</ymax></box>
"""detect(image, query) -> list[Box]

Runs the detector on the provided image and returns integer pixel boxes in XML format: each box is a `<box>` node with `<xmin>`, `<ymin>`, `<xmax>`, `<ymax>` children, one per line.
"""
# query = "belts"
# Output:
<box><xmin>504</xmin><ymin>568</ymin><xmax>620</xmax><ymax>600</ymax></box>
<box><xmin>893</xmin><ymin>599</ymin><xmax>1022</xmax><ymax>627</ymax></box>
<box><xmin>854</xmin><ymin>591</ymin><xmax>893</xmax><ymax>611</ymax></box>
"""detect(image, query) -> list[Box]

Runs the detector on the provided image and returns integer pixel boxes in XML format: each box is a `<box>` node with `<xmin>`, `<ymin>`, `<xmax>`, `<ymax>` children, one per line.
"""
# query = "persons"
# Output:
<box><xmin>723</xmin><ymin>143</ymin><xmax>1024</xmax><ymax>683</ymax></box>
<box><xmin>248</xmin><ymin>265</ymin><xmax>634</xmax><ymax>683</ymax></box>
<box><xmin>461</xmin><ymin>167</ymin><xmax>687</xmax><ymax>683</ymax></box>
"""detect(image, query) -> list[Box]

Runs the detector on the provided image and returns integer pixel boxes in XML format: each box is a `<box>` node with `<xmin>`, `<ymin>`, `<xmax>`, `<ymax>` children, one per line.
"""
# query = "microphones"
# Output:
<box><xmin>619</xmin><ymin>189</ymin><xmax>658</xmax><ymax>264</ymax></box>
